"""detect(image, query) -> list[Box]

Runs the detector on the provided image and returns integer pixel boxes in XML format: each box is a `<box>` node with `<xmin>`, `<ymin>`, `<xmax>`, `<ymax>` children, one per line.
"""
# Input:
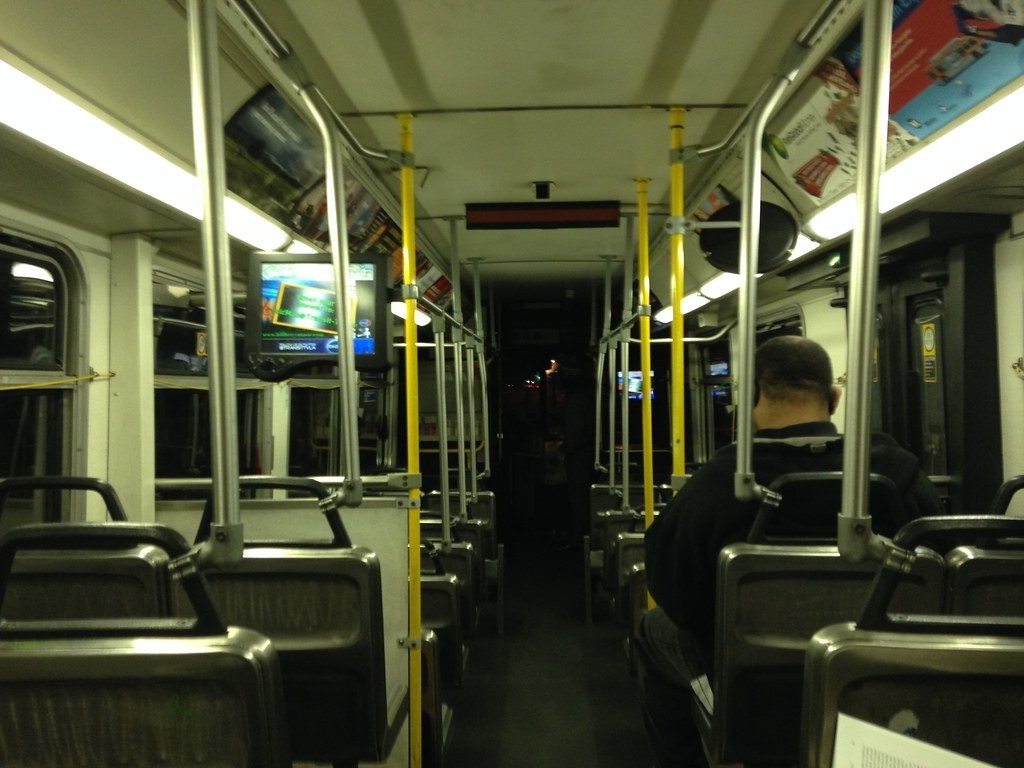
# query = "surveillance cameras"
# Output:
<box><xmin>531</xmin><ymin>182</ymin><xmax>556</xmax><ymax>201</ymax></box>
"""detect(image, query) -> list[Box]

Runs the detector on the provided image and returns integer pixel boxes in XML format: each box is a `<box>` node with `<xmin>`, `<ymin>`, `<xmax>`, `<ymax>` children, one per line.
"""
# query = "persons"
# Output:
<box><xmin>541</xmin><ymin>362</ymin><xmax>595</xmax><ymax>537</ymax></box>
<box><xmin>644</xmin><ymin>337</ymin><xmax>951</xmax><ymax>680</ymax></box>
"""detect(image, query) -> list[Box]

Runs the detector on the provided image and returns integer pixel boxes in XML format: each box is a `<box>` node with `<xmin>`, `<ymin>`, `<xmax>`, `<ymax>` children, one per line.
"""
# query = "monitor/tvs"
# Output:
<box><xmin>245</xmin><ymin>252</ymin><xmax>393</xmax><ymax>383</ymax></box>
<box><xmin>617</xmin><ymin>371</ymin><xmax>655</xmax><ymax>400</ymax></box>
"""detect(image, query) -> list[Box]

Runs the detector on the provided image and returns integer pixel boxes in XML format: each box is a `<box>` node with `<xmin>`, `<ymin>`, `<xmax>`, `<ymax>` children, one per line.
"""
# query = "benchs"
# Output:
<box><xmin>580</xmin><ymin>471</ymin><xmax>1024</xmax><ymax>768</ymax></box>
<box><xmin>0</xmin><ymin>475</ymin><xmax>504</xmax><ymax>768</ymax></box>
